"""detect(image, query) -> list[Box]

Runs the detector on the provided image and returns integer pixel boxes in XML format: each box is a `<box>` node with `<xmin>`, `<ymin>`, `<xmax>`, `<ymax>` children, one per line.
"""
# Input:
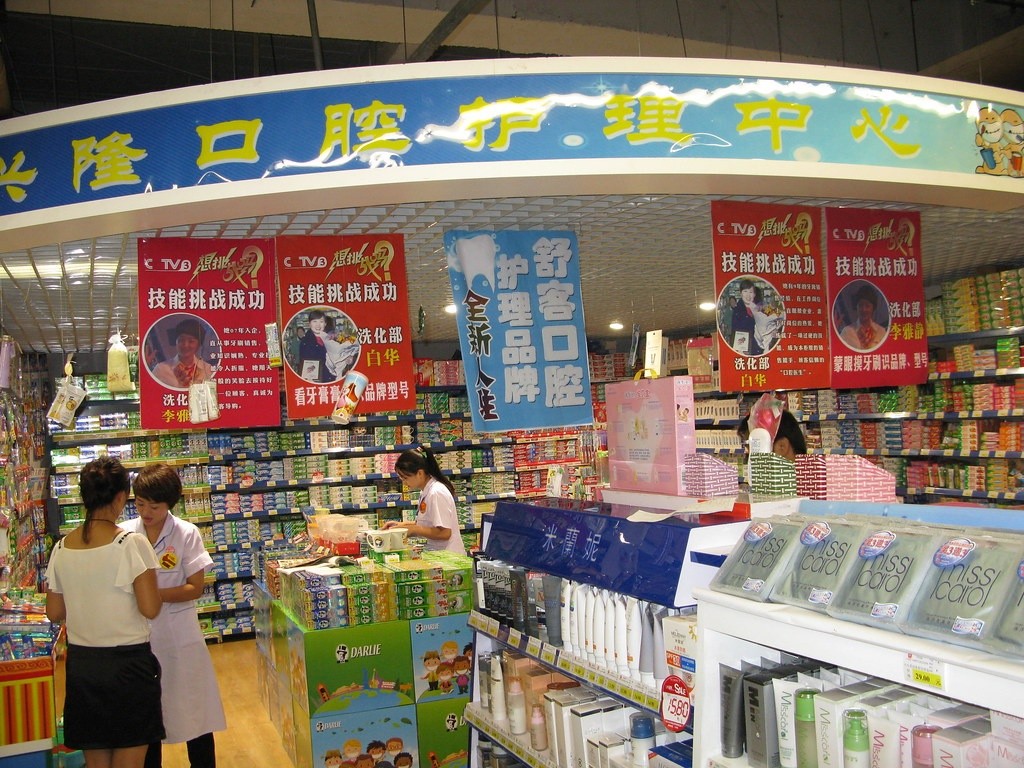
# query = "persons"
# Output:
<box><xmin>839</xmin><ymin>285</ymin><xmax>886</xmax><ymax>350</ymax></box>
<box><xmin>716</xmin><ymin>280</ymin><xmax>783</xmax><ymax>357</ymax></box>
<box><xmin>289</xmin><ymin>309</ymin><xmax>353</xmax><ymax>383</ymax></box>
<box><xmin>381</xmin><ymin>446</ymin><xmax>467</xmax><ymax>558</ymax></box>
<box><xmin>735</xmin><ymin>409</ymin><xmax>807</xmax><ymax>460</ymax></box>
<box><xmin>114</xmin><ymin>459</ymin><xmax>228</xmax><ymax>768</ymax></box>
<box><xmin>44</xmin><ymin>457</ymin><xmax>167</xmax><ymax>768</ymax></box>
<box><xmin>143</xmin><ymin>317</ymin><xmax>214</xmax><ymax>388</ymax></box>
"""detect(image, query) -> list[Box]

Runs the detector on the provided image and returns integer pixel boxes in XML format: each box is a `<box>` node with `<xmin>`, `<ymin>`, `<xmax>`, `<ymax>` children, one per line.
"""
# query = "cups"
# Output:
<box><xmin>367</xmin><ymin>531</ymin><xmax>391</xmax><ymax>553</ymax></box>
<box><xmin>597</xmin><ymin>451</ymin><xmax>609</xmax><ymax>485</ymax></box>
<box><xmin>401</xmin><ymin>435</ymin><xmax>414</xmax><ymax>445</ymax></box>
<box><xmin>331</xmin><ymin>370</ymin><xmax>369</xmax><ymax>425</ymax></box>
<box><xmin>402</xmin><ymin>426</ymin><xmax>414</xmax><ymax>435</ymax></box>
<box><xmin>390</xmin><ymin>528</ymin><xmax>409</xmax><ymax>549</ymax></box>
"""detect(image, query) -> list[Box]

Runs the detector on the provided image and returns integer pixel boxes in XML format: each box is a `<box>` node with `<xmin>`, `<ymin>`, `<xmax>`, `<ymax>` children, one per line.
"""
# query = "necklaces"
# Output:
<box><xmin>89</xmin><ymin>519</ymin><xmax>119</xmax><ymax>528</ymax></box>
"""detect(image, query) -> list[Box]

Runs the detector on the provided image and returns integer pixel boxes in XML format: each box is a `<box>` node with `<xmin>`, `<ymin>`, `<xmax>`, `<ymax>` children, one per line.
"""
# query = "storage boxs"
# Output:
<box><xmin>48</xmin><ymin>265</ymin><xmax>1024</xmax><ymax>768</ymax></box>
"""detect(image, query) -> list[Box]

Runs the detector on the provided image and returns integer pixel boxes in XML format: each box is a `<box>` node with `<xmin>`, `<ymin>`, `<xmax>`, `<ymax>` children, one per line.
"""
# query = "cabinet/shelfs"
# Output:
<box><xmin>1</xmin><ymin>326</ymin><xmax>1024</xmax><ymax>768</ymax></box>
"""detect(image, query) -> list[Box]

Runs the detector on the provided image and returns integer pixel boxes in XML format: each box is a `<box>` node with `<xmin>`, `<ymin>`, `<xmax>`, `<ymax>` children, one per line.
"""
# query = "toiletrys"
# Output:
<box><xmin>717</xmin><ymin>652</ymin><xmax>1024</xmax><ymax>768</ymax></box>
<box><xmin>8</xmin><ymin>585</ymin><xmax>48</xmax><ymax>606</ymax></box>
<box><xmin>476</xmin><ymin>566</ymin><xmax>696</xmax><ymax>768</ymax></box>
<box><xmin>51</xmin><ymin>345</ymin><xmax>641</xmax><ymax>630</ymax></box>
<box><xmin>668</xmin><ymin>268</ymin><xmax>1024</xmax><ymax>494</ymax></box>
